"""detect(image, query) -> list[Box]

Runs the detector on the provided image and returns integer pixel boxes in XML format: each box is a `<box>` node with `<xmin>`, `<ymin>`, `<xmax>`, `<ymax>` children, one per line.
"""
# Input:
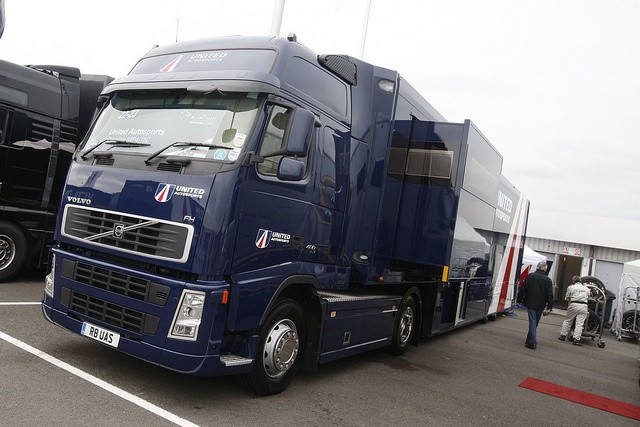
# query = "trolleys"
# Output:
<box><xmin>568</xmin><ymin>285</ymin><xmax>605</xmax><ymax>347</ymax></box>
<box><xmin>611</xmin><ymin>286</ymin><xmax>640</xmax><ymax>343</ymax></box>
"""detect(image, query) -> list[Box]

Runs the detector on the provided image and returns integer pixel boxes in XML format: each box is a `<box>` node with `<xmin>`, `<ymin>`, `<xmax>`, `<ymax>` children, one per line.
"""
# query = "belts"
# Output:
<box><xmin>570</xmin><ymin>301</ymin><xmax>587</xmax><ymax>304</ymax></box>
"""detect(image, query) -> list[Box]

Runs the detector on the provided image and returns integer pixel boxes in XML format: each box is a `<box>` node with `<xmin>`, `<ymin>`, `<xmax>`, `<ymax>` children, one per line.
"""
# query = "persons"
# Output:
<box><xmin>516</xmin><ymin>261</ymin><xmax>553</xmax><ymax>349</ymax></box>
<box><xmin>558</xmin><ymin>276</ymin><xmax>590</xmax><ymax>345</ymax></box>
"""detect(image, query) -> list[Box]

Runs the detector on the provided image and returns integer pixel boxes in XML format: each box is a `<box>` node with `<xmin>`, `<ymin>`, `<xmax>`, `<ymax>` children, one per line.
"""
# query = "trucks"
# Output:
<box><xmin>1</xmin><ymin>1</ymin><xmax>114</xmax><ymax>282</ymax></box>
<box><xmin>41</xmin><ymin>1</ymin><xmax>530</xmax><ymax>396</ymax></box>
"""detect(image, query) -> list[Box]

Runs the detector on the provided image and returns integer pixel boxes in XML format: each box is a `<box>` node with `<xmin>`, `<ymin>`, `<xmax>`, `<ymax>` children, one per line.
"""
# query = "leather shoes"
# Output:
<box><xmin>573</xmin><ymin>339</ymin><xmax>583</xmax><ymax>346</ymax></box>
<box><xmin>558</xmin><ymin>335</ymin><xmax>565</xmax><ymax>340</ymax></box>
<box><xmin>525</xmin><ymin>343</ymin><xmax>536</xmax><ymax>350</ymax></box>
